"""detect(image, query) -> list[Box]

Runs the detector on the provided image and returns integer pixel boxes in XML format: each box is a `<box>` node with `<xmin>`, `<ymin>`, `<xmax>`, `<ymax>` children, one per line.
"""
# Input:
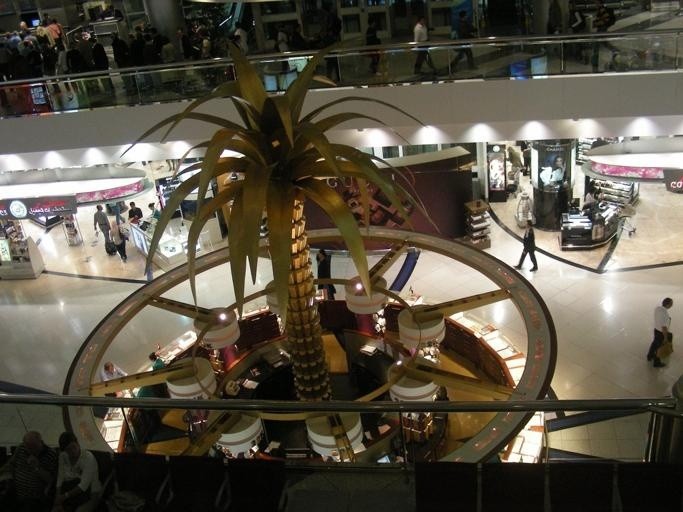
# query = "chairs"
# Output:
<box><xmin>412</xmin><ymin>458</ymin><xmax>683</xmax><ymax>511</ymax></box>
<box><xmin>0</xmin><ymin>445</ymin><xmax>289</xmax><ymax>511</ymax></box>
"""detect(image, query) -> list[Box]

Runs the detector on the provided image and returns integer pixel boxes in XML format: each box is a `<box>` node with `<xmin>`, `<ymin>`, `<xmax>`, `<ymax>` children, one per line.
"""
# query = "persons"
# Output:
<box><xmin>545</xmin><ymin>0</ymin><xmax>562</xmax><ymax>40</ymax></box>
<box><xmin>364</xmin><ymin>18</ymin><xmax>382</xmax><ymax>70</ymax></box>
<box><xmin>99</xmin><ymin>361</ymin><xmax>127</xmax><ymax>398</ymax></box>
<box><xmin>49</xmin><ymin>431</ymin><xmax>101</xmax><ymax>512</ymax></box>
<box><xmin>506</xmin><ymin>147</ymin><xmax>520</xmax><ymax>165</ymax></box>
<box><xmin>645</xmin><ymin>297</ymin><xmax>674</xmax><ymax>369</ymax></box>
<box><xmin>93</xmin><ymin>204</ymin><xmax>112</xmax><ymax>250</ymax></box>
<box><xmin>316</xmin><ymin>249</ymin><xmax>335</xmax><ymax>299</ymax></box>
<box><xmin>567</xmin><ymin>2</ymin><xmax>589</xmax><ymax>65</ymax></box>
<box><xmin>109</xmin><ymin>220</ymin><xmax>128</xmax><ymax>262</ymax></box>
<box><xmin>128</xmin><ymin>201</ymin><xmax>143</xmax><ymax>219</ymax></box>
<box><xmin>109</xmin><ymin>202</ymin><xmax>125</xmax><ymax>225</ymax></box>
<box><xmin>147</xmin><ymin>202</ymin><xmax>160</xmax><ymax>220</ymax></box>
<box><xmin>449</xmin><ymin>10</ymin><xmax>478</xmax><ymax>70</ymax></box>
<box><xmin>550</xmin><ymin>155</ymin><xmax>565</xmax><ymax>181</ymax></box>
<box><xmin>521</xmin><ymin>144</ymin><xmax>531</xmax><ymax>176</ymax></box>
<box><xmin>584</xmin><ymin>187</ymin><xmax>598</xmax><ymax>204</ymax></box>
<box><xmin>513</xmin><ymin>220</ymin><xmax>538</xmax><ymax>271</ymax></box>
<box><xmin>0</xmin><ymin>430</ymin><xmax>56</xmax><ymax>511</ymax></box>
<box><xmin>591</xmin><ymin>1</ymin><xmax>621</xmax><ymax>63</ymax></box>
<box><xmin>148</xmin><ymin>351</ymin><xmax>165</xmax><ymax>370</ymax></box>
<box><xmin>0</xmin><ymin>11</ymin><xmax>342</xmax><ymax>122</ymax></box>
<box><xmin>411</xmin><ymin>17</ymin><xmax>438</xmax><ymax>74</ymax></box>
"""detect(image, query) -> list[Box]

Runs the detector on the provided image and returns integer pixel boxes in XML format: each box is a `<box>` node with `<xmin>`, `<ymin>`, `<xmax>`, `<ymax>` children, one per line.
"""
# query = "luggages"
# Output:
<box><xmin>661</xmin><ymin>318</ymin><xmax>672</xmax><ymax>342</ymax></box>
<box><xmin>105</xmin><ymin>240</ymin><xmax>117</xmax><ymax>255</ymax></box>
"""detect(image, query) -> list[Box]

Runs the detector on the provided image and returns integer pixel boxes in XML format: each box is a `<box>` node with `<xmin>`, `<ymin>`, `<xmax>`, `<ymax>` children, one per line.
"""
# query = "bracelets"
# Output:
<box><xmin>33</xmin><ymin>465</ymin><xmax>41</xmax><ymax>473</ymax></box>
<box><xmin>63</xmin><ymin>491</ymin><xmax>69</xmax><ymax>498</ymax></box>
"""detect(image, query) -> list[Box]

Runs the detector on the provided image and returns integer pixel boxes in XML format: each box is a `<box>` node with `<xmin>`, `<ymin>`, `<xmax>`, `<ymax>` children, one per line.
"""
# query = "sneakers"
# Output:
<box><xmin>653</xmin><ymin>362</ymin><xmax>665</xmax><ymax>368</ymax></box>
<box><xmin>513</xmin><ymin>265</ymin><xmax>538</xmax><ymax>271</ymax></box>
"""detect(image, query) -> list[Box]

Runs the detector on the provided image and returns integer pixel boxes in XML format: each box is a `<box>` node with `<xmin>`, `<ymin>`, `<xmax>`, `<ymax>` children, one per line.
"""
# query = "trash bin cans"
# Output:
<box><xmin>2</xmin><ymin>82</ymin><xmax>50</xmax><ymax>114</ymax></box>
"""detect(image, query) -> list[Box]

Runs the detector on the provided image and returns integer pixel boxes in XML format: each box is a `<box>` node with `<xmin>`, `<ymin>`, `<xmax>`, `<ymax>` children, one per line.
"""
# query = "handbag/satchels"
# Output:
<box><xmin>118</xmin><ymin>227</ymin><xmax>130</xmax><ymax>240</ymax></box>
<box><xmin>657</xmin><ymin>342</ymin><xmax>674</xmax><ymax>357</ymax></box>
<box><xmin>60</xmin><ymin>478</ymin><xmax>91</xmax><ymax>509</ymax></box>
